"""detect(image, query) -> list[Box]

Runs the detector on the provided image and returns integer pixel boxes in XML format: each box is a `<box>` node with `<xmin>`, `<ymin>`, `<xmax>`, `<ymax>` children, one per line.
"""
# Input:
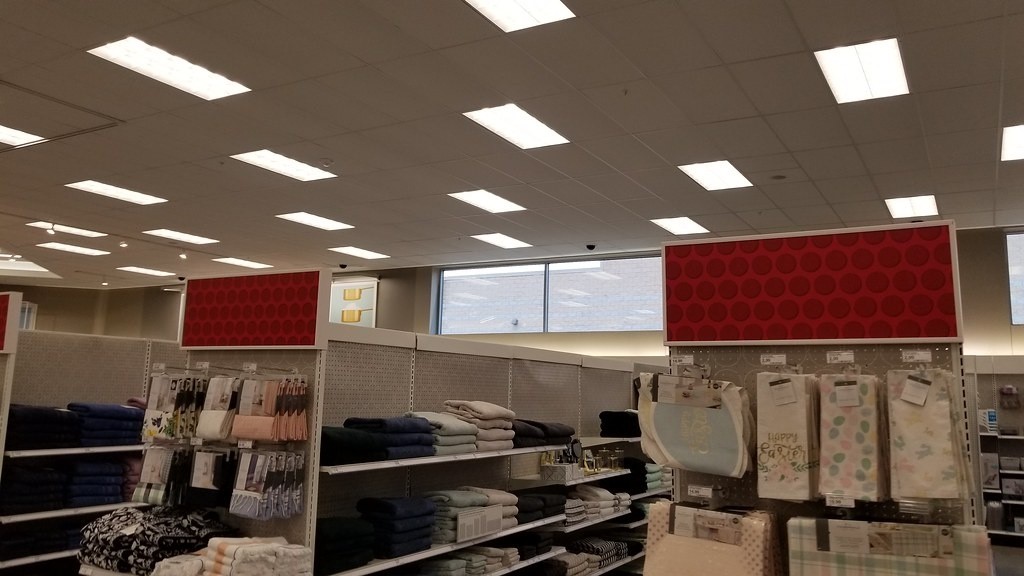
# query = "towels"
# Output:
<box><xmin>756</xmin><ymin>369</ymin><xmax>975</xmax><ymax>503</ymax></box>
<box><xmin>315</xmin><ymin>400</ymin><xmax>673</xmax><ymax>575</ymax></box>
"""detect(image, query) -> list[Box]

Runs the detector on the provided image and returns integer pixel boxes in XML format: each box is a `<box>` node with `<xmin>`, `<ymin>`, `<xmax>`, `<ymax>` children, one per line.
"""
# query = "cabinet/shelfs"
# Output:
<box><xmin>0</xmin><ymin>218</ymin><xmax>1024</xmax><ymax>576</ymax></box>
